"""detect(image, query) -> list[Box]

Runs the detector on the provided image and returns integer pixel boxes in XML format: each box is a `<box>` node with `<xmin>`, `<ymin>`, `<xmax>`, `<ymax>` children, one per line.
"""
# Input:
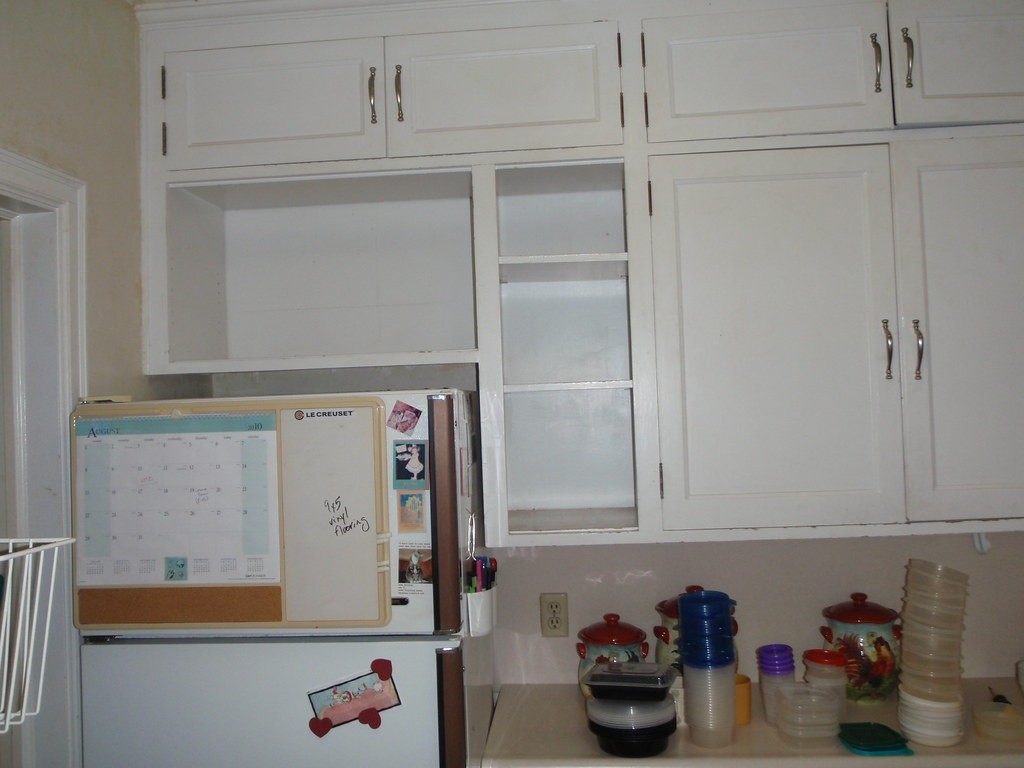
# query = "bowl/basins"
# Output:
<box><xmin>587</xmin><ymin>693</ymin><xmax>677</xmax><ymax>758</ymax></box>
<box><xmin>897</xmin><ymin>558</ymin><xmax>968</xmax><ymax>748</ymax></box>
<box><xmin>1017</xmin><ymin>661</ymin><xmax>1023</xmax><ymax>693</ymax></box>
<box><xmin>579</xmin><ymin>661</ymin><xmax>678</xmax><ymax>700</ymax></box>
<box><xmin>971</xmin><ymin>700</ymin><xmax>1023</xmax><ymax>741</ymax></box>
<box><xmin>777</xmin><ymin>681</ymin><xmax>842</xmax><ymax>751</ymax></box>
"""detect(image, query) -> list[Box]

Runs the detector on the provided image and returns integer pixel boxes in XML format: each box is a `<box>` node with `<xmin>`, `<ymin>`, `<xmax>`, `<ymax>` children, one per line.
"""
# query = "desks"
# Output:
<box><xmin>478</xmin><ymin>674</ymin><xmax>1024</xmax><ymax>768</ymax></box>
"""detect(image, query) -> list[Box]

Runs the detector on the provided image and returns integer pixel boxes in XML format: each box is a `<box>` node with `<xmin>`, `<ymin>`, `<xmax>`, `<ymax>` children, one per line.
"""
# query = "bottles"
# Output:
<box><xmin>576</xmin><ymin>613</ymin><xmax>649</xmax><ymax>700</ymax></box>
<box><xmin>819</xmin><ymin>592</ymin><xmax>903</xmax><ymax>705</ymax></box>
<box><xmin>654</xmin><ymin>583</ymin><xmax>739</xmax><ymax>689</ymax></box>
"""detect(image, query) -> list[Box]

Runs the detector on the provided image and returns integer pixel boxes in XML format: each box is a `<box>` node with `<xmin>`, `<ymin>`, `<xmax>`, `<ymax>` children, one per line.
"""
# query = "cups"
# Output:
<box><xmin>755</xmin><ymin>642</ymin><xmax>795</xmax><ymax>724</ymax></box>
<box><xmin>679</xmin><ymin>591</ymin><xmax>736</xmax><ymax>747</ymax></box>
<box><xmin>802</xmin><ymin>648</ymin><xmax>848</xmax><ymax>721</ymax></box>
<box><xmin>735</xmin><ymin>675</ymin><xmax>751</xmax><ymax>724</ymax></box>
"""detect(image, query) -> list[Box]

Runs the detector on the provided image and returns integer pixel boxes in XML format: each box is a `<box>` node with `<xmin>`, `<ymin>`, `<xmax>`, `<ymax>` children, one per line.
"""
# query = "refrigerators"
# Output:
<box><xmin>70</xmin><ymin>391</ymin><xmax>495</xmax><ymax>767</ymax></box>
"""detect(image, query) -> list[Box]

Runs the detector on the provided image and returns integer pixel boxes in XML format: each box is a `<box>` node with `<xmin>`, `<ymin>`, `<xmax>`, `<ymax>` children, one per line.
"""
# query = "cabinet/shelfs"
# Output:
<box><xmin>128</xmin><ymin>0</ymin><xmax>1019</xmax><ymax>550</ymax></box>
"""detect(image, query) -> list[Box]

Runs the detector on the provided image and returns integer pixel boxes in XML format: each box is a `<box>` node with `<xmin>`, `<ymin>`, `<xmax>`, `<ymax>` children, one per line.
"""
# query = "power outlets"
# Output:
<box><xmin>538</xmin><ymin>593</ymin><xmax>570</xmax><ymax>638</ymax></box>
